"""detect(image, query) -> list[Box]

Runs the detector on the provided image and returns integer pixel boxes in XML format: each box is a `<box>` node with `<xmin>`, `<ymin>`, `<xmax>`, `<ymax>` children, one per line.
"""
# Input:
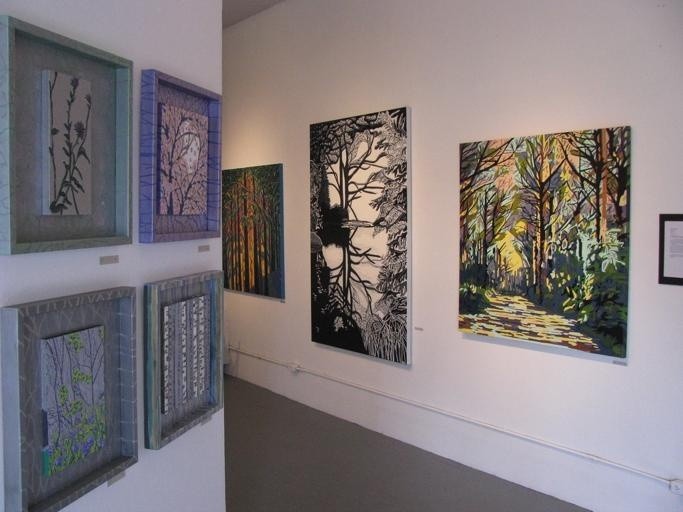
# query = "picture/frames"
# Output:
<box><xmin>139</xmin><ymin>69</ymin><xmax>221</xmax><ymax>244</ymax></box>
<box><xmin>0</xmin><ymin>286</ymin><xmax>138</xmax><ymax>512</ymax></box>
<box><xmin>659</xmin><ymin>213</ymin><xmax>683</xmax><ymax>285</ymax></box>
<box><xmin>0</xmin><ymin>16</ymin><xmax>133</xmax><ymax>257</ymax></box>
<box><xmin>144</xmin><ymin>271</ymin><xmax>224</xmax><ymax>449</ymax></box>
